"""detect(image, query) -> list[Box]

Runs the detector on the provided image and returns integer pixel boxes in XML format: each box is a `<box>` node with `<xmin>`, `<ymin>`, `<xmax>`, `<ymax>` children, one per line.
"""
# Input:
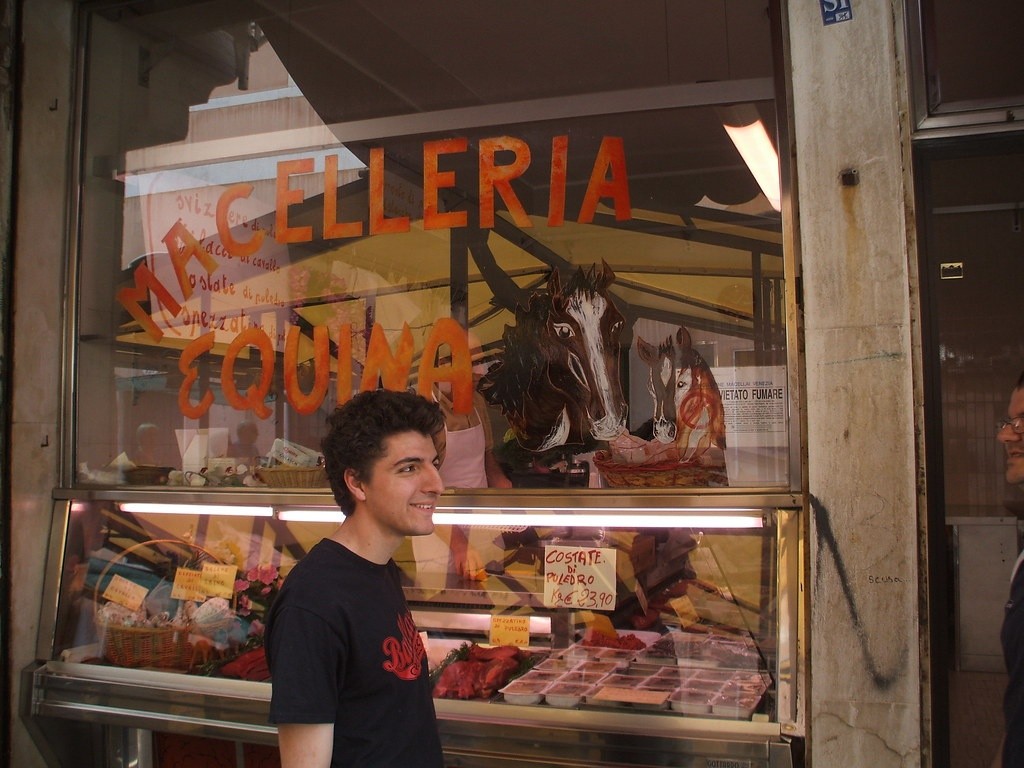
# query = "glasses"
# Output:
<box><xmin>994</xmin><ymin>414</ymin><xmax>1024</xmax><ymax>435</ymax></box>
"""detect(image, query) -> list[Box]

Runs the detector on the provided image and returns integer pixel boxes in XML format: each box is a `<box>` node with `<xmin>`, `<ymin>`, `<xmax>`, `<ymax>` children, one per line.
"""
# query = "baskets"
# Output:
<box><xmin>94</xmin><ymin>539</ymin><xmax>237</xmax><ymax>671</ymax></box>
<box><xmin>251</xmin><ymin>452</ymin><xmax>327</xmax><ymax>489</ymax></box>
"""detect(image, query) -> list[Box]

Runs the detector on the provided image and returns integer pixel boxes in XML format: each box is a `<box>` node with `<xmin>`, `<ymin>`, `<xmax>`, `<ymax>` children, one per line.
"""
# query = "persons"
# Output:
<box><xmin>995</xmin><ymin>376</ymin><xmax>1023</xmax><ymax>767</ymax></box>
<box><xmin>206</xmin><ymin>418</ymin><xmax>261</xmax><ymax>470</ymax></box>
<box><xmin>594</xmin><ymin>531</ymin><xmax>702</xmax><ymax>767</ymax></box>
<box><xmin>410</xmin><ymin>327</ymin><xmax>512</xmax><ymax>595</ymax></box>
<box><xmin>129</xmin><ymin>422</ymin><xmax>169</xmax><ymax>466</ymax></box>
<box><xmin>262</xmin><ymin>388</ymin><xmax>449</xmax><ymax>767</ymax></box>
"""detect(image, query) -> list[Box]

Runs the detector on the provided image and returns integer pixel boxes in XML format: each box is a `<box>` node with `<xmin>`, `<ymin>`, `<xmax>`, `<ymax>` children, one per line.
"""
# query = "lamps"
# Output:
<box><xmin>113</xmin><ymin>501</ymin><xmax>770</xmax><ymax>528</ymax></box>
<box><xmin>714</xmin><ymin>101</ymin><xmax>781</xmax><ymax>214</ymax></box>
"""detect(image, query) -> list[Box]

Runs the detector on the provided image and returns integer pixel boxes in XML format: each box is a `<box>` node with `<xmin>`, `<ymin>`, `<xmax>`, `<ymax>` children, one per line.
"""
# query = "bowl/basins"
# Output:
<box><xmin>122</xmin><ymin>466</ymin><xmax>176</xmax><ymax>486</ymax></box>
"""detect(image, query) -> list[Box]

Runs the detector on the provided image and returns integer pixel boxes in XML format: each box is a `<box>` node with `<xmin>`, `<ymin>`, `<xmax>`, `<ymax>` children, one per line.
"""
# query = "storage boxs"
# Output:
<box><xmin>497</xmin><ymin>645</ymin><xmax>773</xmax><ymax>718</ymax></box>
